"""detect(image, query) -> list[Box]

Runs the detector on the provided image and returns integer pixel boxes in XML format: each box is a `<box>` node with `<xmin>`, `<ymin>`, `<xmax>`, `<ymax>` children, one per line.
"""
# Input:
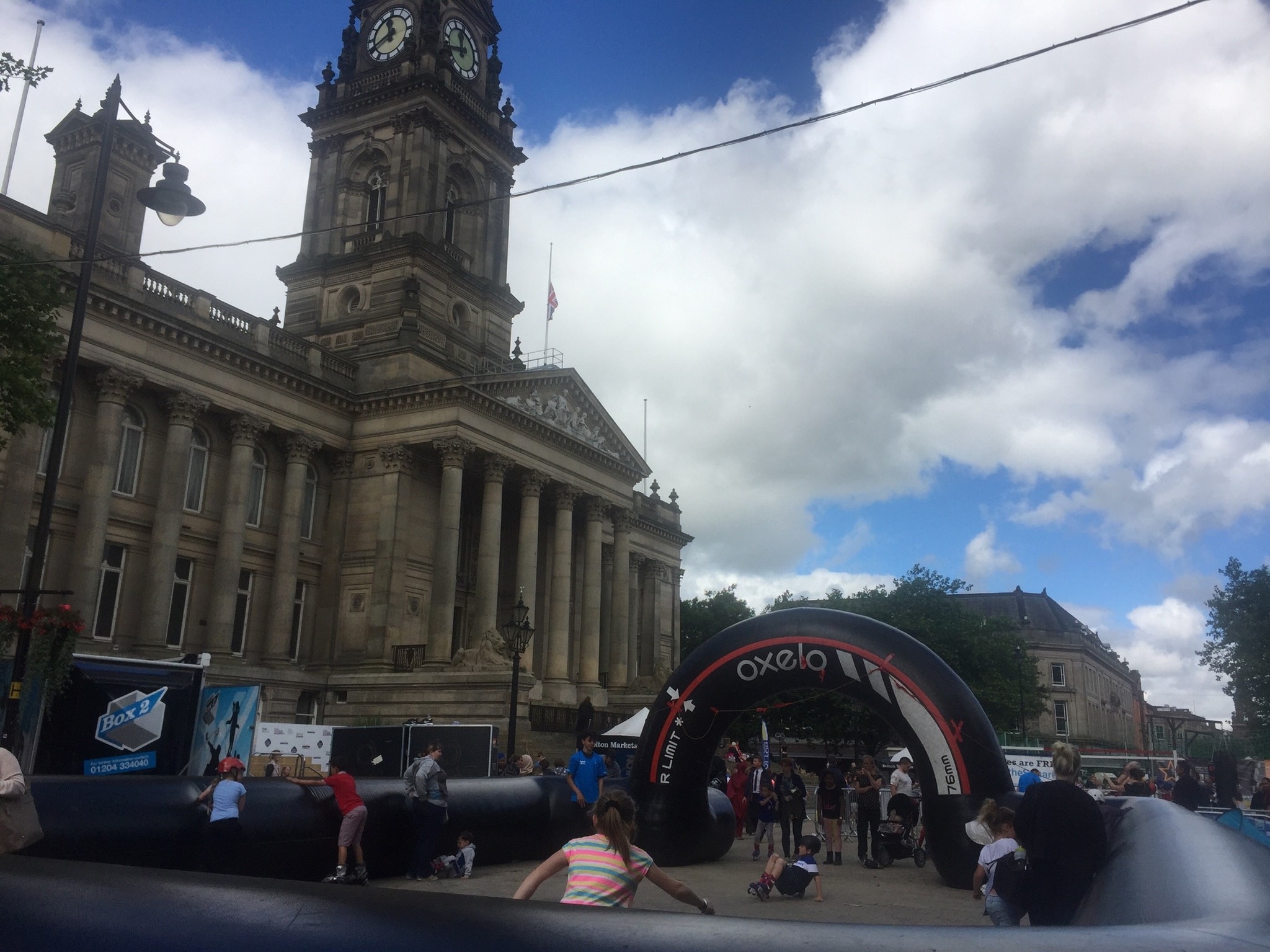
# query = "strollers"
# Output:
<box><xmin>874</xmin><ymin>790</ymin><xmax>927</xmax><ymax>868</ymax></box>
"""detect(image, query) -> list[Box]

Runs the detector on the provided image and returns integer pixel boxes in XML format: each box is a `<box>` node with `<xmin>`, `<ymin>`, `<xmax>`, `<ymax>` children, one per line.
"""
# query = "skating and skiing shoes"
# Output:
<box><xmin>747</xmin><ymin>883</ymin><xmax>769</xmax><ymax>902</ymax></box>
<box><xmin>322</xmin><ymin>864</ymin><xmax>347</xmax><ymax>884</ymax></box>
<box><xmin>767</xmin><ymin>851</ymin><xmax>774</xmax><ymax>859</ymax></box>
<box><xmin>345</xmin><ymin>863</ymin><xmax>368</xmax><ymax>885</ymax></box>
<box><xmin>752</xmin><ymin>850</ymin><xmax>760</xmax><ymax>860</ymax></box>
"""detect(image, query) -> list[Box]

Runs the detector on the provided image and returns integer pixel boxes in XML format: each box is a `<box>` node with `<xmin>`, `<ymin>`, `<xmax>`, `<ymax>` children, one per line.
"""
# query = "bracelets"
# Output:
<box><xmin>701</xmin><ymin>898</ymin><xmax>709</xmax><ymax>913</ymax></box>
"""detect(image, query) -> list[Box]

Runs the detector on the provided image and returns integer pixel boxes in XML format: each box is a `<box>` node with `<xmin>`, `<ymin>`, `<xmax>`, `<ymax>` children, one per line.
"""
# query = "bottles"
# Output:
<box><xmin>1014</xmin><ymin>846</ymin><xmax>1026</xmax><ymax>860</ymax></box>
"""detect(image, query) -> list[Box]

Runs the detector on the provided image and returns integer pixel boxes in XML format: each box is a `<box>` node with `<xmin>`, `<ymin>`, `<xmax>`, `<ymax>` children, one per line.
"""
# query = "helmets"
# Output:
<box><xmin>218</xmin><ymin>757</ymin><xmax>246</xmax><ymax>774</ymax></box>
<box><xmin>800</xmin><ymin>835</ymin><xmax>821</xmax><ymax>853</ymax></box>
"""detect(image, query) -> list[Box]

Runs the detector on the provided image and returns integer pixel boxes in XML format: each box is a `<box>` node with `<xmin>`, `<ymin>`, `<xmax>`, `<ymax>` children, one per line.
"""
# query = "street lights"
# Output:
<box><xmin>0</xmin><ymin>74</ymin><xmax>206</xmax><ymax>757</ymax></box>
<box><xmin>500</xmin><ymin>586</ymin><xmax>537</xmax><ymax>766</ymax></box>
<box><xmin>1012</xmin><ymin>646</ymin><xmax>1029</xmax><ymax>747</ymax></box>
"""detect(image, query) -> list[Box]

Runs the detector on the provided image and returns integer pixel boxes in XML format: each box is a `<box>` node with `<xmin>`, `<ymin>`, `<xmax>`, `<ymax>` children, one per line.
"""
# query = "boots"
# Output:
<box><xmin>823</xmin><ymin>851</ymin><xmax>833</xmax><ymax>864</ymax></box>
<box><xmin>833</xmin><ymin>851</ymin><xmax>842</xmax><ymax>865</ymax></box>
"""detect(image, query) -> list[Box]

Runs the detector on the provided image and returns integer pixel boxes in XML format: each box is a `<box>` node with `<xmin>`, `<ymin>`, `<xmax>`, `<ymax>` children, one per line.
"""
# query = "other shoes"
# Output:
<box><xmin>406</xmin><ymin>873</ymin><xmax>438</xmax><ymax>881</ymax></box>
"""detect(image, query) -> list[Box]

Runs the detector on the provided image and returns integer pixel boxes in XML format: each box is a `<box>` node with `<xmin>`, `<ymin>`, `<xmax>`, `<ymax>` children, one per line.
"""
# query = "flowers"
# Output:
<box><xmin>0</xmin><ymin>603</ymin><xmax>87</xmax><ymax>725</ymax></box>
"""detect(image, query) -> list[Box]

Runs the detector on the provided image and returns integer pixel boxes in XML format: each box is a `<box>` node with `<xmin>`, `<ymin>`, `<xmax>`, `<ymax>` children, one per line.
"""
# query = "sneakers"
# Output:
<box><xmin>402</xmin><ymin>718</ymin><xmax>418</xmax><ymax>726</ymax></box>
<box><xmin>417</xmin><ymin>718</ymin><xmax>433</xmax><ymax>725</ymax></box>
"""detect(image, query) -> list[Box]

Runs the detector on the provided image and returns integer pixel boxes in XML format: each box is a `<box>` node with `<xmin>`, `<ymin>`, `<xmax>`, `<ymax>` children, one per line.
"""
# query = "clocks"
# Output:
<box><xmin>443</xmin><ymin>15</ymin><xmax>481</xmax><ymax>82</ymax></box>
<box><xmin>367</xmin><ymin>7</ymin><xmax>415</xmax><ymax>63</ymax></box>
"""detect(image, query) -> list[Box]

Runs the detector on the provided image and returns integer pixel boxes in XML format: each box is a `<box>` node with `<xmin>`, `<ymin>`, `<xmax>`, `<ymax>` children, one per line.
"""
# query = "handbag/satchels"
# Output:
<box><xmin>985</xmin><ymin>848</ymin><xmax>1028</xmax><ymax>903</ymax></box>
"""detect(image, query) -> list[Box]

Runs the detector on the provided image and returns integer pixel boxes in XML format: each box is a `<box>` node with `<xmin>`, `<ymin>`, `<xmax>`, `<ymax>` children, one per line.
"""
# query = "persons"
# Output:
<box><xmin>726</xmin><ymin>741</ymin><xmax>1270</xmax><ymax>928</ymax></box>
<box><xmin>0</xmin><ymin>748</ymin><xmax>43</xmax><ymax>855</ymax></box>
<box><xmin>266</xmin><ymin>742</ymin><xmax>715</xmax><ymax>915</ymax></box>
<box><xmin>196</xmin><ymin>758</ymin><xmax>247</xmax><ymax>875</ymax></box>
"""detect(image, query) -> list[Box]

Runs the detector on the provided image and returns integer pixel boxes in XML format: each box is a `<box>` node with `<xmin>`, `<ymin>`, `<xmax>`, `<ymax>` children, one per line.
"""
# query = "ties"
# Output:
<box><xmin>754</xmin><ymin>770</ymin><xmax>759</xmax><ymax>791</ymax></box>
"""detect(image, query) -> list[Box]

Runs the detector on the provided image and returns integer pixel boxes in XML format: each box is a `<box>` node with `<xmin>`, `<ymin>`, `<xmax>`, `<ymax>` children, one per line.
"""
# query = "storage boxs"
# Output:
<box><xmin>328</xmin><ymin>724</ymin><xmax>500</xmax><ymax>779</ymax></box>
